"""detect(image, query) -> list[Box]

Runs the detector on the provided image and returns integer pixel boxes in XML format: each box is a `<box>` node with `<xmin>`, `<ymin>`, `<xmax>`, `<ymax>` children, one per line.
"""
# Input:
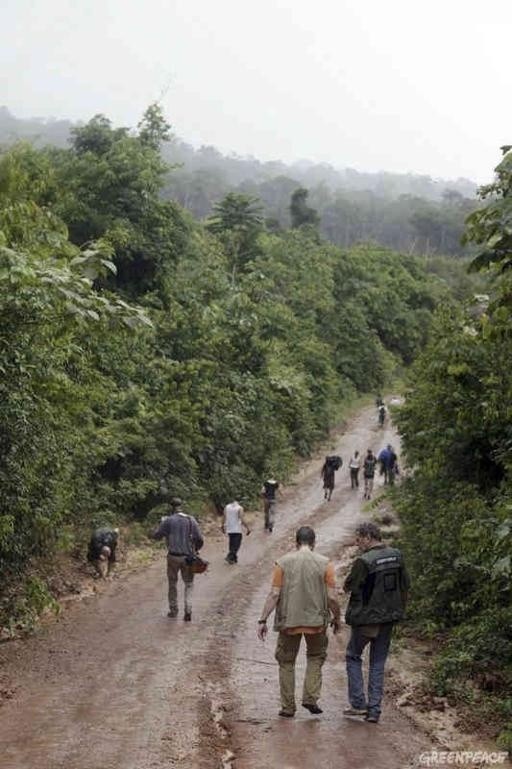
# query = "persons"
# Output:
<box><xmin>257</xmin><ymin>525</ymin><xmax>342</xmax><ymax>717</ymax></box>
<box><xmin>148</xmin><ymin>497</ymin><xmax>204</xmax><ymax>621</ymax></box>
<box><xmin>87</xmin><ymin>526</ymin><xmax>119</xmax><ymax>564</ymax></box>
<box><xmin>342</xmin><ymin>521</ymin><xmax>412</xmax><ymax>723</ymax></box>
<box><xmin>363</xmin><ymin>449</ymin><xmax>377</xmax><ymax>500</ymax></box>
<box><xmin>377</xmin><ymin>444</ymin><xmax>397</xmax><ymax>486</ymax></box>
<box><xmin>348</xmin><ymin>451</ymin><xmax>360</xmax><ymax>489</ymax></box>
<box><xmin>219</xmin><ymin>495</ymin><xmax>252</xmax><ymax>565</ymax></box>
<box><xmin>379</xmin><ymin>407</ymin><xmax>385</xmax><ymax>426</ymax></box>
<box><xmin>260</xmin><ymin>474</ymin><xmax>283</xmax><ymax>533</ymax></box>
<box><xmin>321</xmin><ymin>448</ymin><xmax>343</xmax><ymax>501</ymax></box>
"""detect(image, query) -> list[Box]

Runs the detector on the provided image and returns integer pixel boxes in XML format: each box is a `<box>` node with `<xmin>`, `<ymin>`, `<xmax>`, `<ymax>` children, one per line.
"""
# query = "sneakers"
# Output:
<box><xmin>302</xmin><ymin>703</ymin><xmax>322</xmax><ymax>713</ymax></box>
<box><xmin>279</xmin><ymin>709</ymin><xmax>294</xmax><ymax>719</ymax></box>
<box><xmin>344</xmin><ymin>707</ymin><xmax>378</xmax><ymax>722</ymax></box>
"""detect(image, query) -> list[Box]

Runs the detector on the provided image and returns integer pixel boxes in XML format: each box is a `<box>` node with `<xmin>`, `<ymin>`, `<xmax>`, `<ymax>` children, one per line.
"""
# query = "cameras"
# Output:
<box><xmin>185</xmin><ymin>556</ymin><xmax>196</xmax><ymax>565</ymax></box>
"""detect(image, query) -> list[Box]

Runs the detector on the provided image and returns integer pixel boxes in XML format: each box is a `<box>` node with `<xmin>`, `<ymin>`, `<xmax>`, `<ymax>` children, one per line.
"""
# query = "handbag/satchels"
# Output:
<box><xmin>190</xmin><ymin>558</ymin><xmax>208</xmax><ymax>573</ymax></box>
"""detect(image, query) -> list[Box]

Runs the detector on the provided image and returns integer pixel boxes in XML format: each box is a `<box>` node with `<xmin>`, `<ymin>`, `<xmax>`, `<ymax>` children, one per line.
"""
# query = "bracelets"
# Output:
<box><xmin>258</xmin><ymin>620</ymin><xmax>266</xmax><ymax>624</ymax></box>
